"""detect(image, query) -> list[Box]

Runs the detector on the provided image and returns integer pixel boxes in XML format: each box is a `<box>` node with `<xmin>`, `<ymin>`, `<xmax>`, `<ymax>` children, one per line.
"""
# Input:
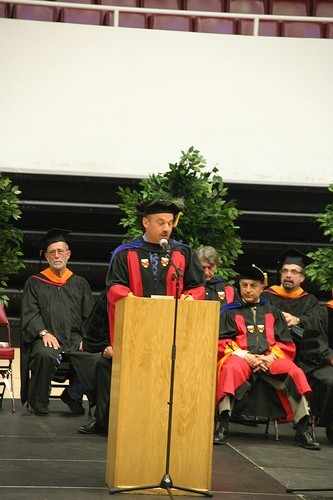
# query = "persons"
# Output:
<box><xmin>262</xmin><ymin>247</ymin><xmax>333</xmax><ymax>442</ymax></box>
<box><xmin>213</xmin><ymin>257</ymin><xmax>320</xmax><ymax>449</ymax></box>
<box><xmin>77</xmin><ymin>269</ymin><xmax>112</xmax><ymax>435</ymax></box>
<box><xmin>194</xmin><ymin>245</ymin><xmax>240</xmax><ymax>307</ymax></box>
<box><xmin>106</xmin><ymin>201</ymin><xmax>206</xmax><ymax>349</ymax></box>
<box><xmin>20</xmin><ymin>228</ymin><xmax>96</xmax><ymax>414</ymax></box>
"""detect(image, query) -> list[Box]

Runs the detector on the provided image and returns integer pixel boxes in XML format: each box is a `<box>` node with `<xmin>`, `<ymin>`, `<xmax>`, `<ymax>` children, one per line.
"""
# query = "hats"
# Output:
<box><xmin>135</xmin><ymin>199</ymin><xmax>183</xmax><ymax>215</ymax></box>
<box><xmin>237</xmin><ymin>264</ymin><xmax>265</xmax><ymax>281</ymax></box>
<box><xmin>31</xmin><ymin>228</ymin><xmax>72</xmax><ymax>252</ymax></box>
<box><xmin>275</xmin><ymin>248</ymin><xmax>316</xmax><ymax>269</ymax></box>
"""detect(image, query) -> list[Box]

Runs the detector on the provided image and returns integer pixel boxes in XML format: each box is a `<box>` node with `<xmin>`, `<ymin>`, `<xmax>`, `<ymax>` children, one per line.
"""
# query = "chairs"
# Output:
<box><xmin>0</xmin><ymin>304</ymin><xmax>333</xmax><ymax>441</ymax></box>
<box><xmin>0</xmin><ymin>0</ymin><xmax>333</xmax><ymax>39</ymax></box>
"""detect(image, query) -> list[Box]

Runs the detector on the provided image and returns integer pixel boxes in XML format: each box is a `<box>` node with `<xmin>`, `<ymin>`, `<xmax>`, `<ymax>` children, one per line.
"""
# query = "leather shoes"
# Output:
<box><xmin>294</xmin><ymin>432</ymin><xmax>321</xmax><ymax>448</ymax></box>
<box><xmin>213</xmin><ymin>427</ymin><xmax>230</xmax><ymax>444</ymax></box>
<box><xmin>77</xmin><ymin>420</ymin><xmax>99</xmax><ymax>433</ymax></box>
<box><xmin>231</xmin><ymin>414</ymin><xmax>256</xmax><ymax>425</ymax></box>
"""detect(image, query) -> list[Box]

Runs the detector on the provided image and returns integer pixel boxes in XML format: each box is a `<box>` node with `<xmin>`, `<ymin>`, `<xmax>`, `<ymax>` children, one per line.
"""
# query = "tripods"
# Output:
<box><xmin>109</xmin><ymin>247</ymin><xmax>214</xmax><ymax>500</ymax></box>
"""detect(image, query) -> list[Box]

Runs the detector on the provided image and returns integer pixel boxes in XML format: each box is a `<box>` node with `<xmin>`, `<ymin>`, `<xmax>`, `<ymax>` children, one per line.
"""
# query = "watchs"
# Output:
<box><xmin>41</xmin><ymin>330</ymin><xmax>50</xmax><ymax>336</ymax></box>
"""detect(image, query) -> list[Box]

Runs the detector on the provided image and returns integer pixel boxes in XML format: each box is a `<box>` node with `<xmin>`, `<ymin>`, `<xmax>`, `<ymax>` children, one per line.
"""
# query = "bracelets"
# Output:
<box><xmin>297</xmin><ymin>316</ymin><xmax>300</xmax><ymax>322</ymax></box>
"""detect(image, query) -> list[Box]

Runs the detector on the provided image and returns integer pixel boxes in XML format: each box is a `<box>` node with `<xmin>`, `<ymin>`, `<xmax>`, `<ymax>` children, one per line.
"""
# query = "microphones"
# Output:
<box><xmin>159</xmin><ymin>239</ymin><xmax>170</xmax><ymax>250</ymax></box>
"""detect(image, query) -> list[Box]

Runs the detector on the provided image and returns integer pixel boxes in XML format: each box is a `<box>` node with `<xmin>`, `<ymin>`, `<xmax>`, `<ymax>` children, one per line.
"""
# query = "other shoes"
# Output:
<box><xmin>30</xmin><ymin>405</ymin><xmax>49</xmax><ymax>413</ymax></box>
<box><xmin>60</xmin><ymin>389</ymin><xmax>85</xmax><ymax>414</ymax></box>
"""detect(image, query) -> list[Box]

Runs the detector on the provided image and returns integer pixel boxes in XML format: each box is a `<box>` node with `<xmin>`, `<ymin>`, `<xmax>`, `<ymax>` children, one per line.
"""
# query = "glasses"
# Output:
<box><xmin>279</xmin><ymin>268</ymin><xmax>303</xmax><ymax>275</ymax></box>
